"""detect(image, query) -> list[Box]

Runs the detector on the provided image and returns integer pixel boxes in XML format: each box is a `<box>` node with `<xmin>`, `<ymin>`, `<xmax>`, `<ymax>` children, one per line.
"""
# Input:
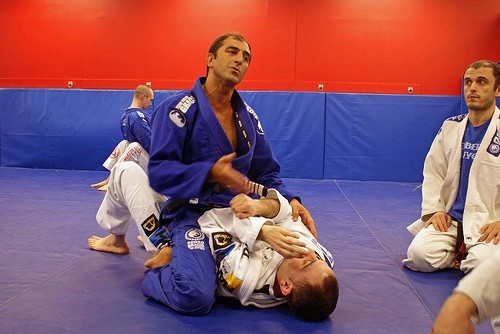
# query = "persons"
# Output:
<box><xmin>88</xmin><ymin>160</ymin><xmax>338</xmax><ymax>321</ymax></box>
<box><xmin>402</xmin><ymin>60</ymin><xmax>500</xmax><ymax>273</ymax></box>
<box><xmin>119</xmin><ymin>85</ymin><xmax>155</xmax><ymax>154</ymax></box>
<box><xmin>91</xmin><ymin>140</ymin><xmax>150</xmax><ymax>191</ymax></box>
<box><xmin>140</xmin><ymin>34</ymin><xmax>318</xmax><ymax>315</ymax></box>
<box><xmin>431</xmin><ymin>246</ymin><xmax>500</xmax><ymax>334</ymax></box>
